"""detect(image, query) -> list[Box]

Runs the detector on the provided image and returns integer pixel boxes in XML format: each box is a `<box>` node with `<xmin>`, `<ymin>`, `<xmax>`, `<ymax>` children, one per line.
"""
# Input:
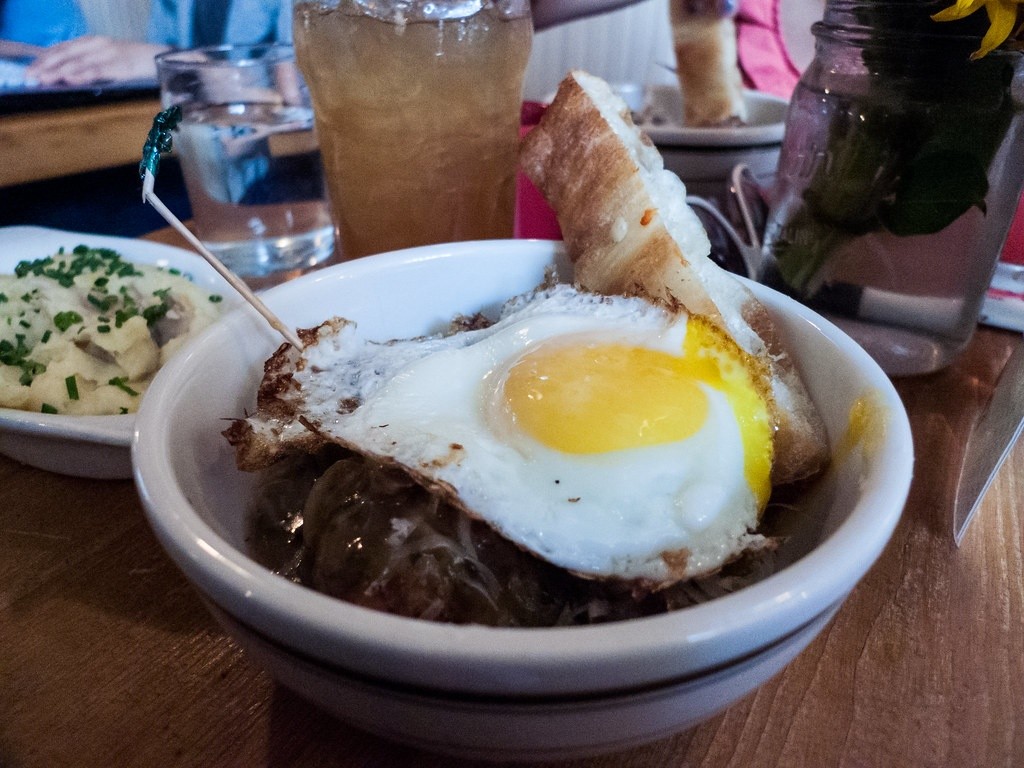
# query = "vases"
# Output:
<box><xmin>752</xmin><ymin>0</ymin><xmax>1024</xmax><ymax>373</ymax></box>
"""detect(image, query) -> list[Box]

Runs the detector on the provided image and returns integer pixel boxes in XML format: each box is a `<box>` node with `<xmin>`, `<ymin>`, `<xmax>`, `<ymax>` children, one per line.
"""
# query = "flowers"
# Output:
<box><xmin>766</xmin><ymin>0</ymin><xmax>1023</xmax><ymax>300</ymax></box>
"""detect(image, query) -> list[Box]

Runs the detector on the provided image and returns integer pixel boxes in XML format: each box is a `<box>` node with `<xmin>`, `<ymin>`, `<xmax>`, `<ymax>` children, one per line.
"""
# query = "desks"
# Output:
<box><xmin>1</xmin><ymin>95</ymin><xmax>1024</xmax><ymax>767</ymax></box>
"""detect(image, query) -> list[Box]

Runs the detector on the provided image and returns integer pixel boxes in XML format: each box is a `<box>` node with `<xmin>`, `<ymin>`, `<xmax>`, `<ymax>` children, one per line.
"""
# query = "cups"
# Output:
<box><xmin>138</xmin><ymin>0</ymin><xmax>534</xmax><ymax>278</ymax></box>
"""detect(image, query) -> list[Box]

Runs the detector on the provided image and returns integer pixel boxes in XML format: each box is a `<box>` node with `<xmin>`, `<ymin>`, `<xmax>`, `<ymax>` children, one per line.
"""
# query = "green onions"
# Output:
<box><xmin>0</xmin><ymin>242</ymin><xmax>224</xmax><ymax>419</ymax></box>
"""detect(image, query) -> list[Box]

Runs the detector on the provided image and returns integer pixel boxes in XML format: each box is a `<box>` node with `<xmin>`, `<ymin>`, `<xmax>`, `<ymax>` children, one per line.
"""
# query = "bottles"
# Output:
<box><xmin>758</xmin><ymin>1</ymin><xmax>1024</xmax><ymax>376</ymax></box>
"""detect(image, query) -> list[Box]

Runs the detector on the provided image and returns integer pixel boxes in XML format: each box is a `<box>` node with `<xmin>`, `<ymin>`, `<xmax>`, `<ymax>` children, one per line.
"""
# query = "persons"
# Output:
<box><xmin>20</xmin><ymin>0</ymin><xmax>317</xmax><ymax>109</ymax></box>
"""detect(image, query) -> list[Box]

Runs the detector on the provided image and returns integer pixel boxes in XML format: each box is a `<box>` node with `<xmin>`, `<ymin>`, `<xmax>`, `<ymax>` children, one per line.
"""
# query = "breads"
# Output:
<box><xmin>518</xmin><ymin>70</ymin><xmax>829</xmax><ymax>493</ymax></box>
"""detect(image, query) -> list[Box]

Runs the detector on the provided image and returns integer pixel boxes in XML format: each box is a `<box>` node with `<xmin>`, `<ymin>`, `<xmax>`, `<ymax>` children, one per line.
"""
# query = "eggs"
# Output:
<box><xmin>286</xmin><ymin>292</ymin><xmax>779</xmax><ymax>586</ymax></box>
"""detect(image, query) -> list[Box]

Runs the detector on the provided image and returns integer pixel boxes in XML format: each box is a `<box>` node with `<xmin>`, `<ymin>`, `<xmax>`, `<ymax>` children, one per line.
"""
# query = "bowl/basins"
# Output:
<box><xmin>618</xmin><ymin>83</ymin><xmax>790</xmax><ymax>185</ymax></box>
<box><xmin>0</xmin><ymin>226</ymin><xmax>251</xmax><ymax>479</ymax></box>
<box><xmin>133</xmin><ymin>239</ymin><xmax>914</xmax><ymax>763</ymax></box>
